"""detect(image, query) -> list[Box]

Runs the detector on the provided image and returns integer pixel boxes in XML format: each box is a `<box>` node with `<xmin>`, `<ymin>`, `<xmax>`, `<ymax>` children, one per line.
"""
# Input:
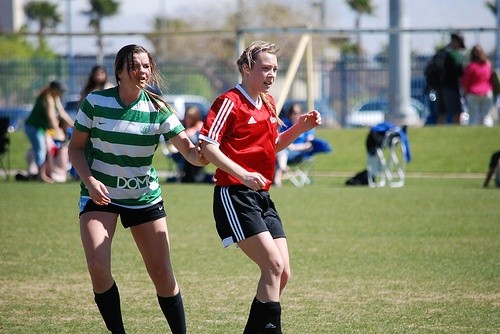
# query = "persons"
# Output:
<box><xmin>423</xmin><ymin>32</ymin><xmax>472</xmax><ymax>127</ymax></box>
<box><xmin>162</xmin><ymin>107</ymin><xmax>204</xmax><ymax>156</ymax></box>
<box><xmin>68</xmin><ymin>43</ymin><xmax>210</xmax><ymax>334</ymax></box>
<box><xmin>78</xmin><ymin>64</ymin><xmax>115</xmax><ymax>111</ymax></box>
<box><xmin>274</xmin><ymin>103</ymin><xmax>314</xmax><ymax>187</ymax></box>
<box><xmin>24</xmin><ymin>80</ymin><xmax>82</xmax><ymax>183</ymax></box>
<box><xmin>462</xmin><ymin>45</ymin><xmax>492</xmax><ymax>126</ymax></box>
<box><xmin>198</xmin><ymin>41</ymin><xmax>322</xmax><ymax>334</ymax></box>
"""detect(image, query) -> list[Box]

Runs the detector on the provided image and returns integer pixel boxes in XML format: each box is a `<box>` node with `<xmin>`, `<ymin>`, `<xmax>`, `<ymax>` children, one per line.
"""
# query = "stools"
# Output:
<box><xmin>0</xmin><ymin>117</ymin><xmax>404</xmax><ymax>187</ymax></box>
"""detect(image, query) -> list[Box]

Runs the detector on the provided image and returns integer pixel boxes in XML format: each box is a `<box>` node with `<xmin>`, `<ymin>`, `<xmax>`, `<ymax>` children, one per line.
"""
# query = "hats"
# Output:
<box><xmin>51</xmin><ymin>80</ymin><xmax>66</xmax><ymax>92</ymax></box>
<box><xmin>452</xmin><ymin>33</ymin><xmax>465</xmax><ymax>48</ymax></box>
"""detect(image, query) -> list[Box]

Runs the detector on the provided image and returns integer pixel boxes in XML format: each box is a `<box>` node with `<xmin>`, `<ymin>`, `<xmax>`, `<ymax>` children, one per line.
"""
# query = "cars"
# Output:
<box><xmin>0</xmin><ymin>109</ymin><xmax>20</xmax><ymax>133</ymax></box>
<box><xmin>344</xmin><ymin>98</ymin><xmax>426</xmax><ymax>125</ymax></box>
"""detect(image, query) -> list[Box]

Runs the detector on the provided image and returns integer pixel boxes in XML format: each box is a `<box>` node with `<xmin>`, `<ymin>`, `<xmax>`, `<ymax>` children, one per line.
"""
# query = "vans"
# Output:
<box><xmin>157</xmin><ymin>94</ymin><xmax>212</xmax><ymax>125</ymax></box>
